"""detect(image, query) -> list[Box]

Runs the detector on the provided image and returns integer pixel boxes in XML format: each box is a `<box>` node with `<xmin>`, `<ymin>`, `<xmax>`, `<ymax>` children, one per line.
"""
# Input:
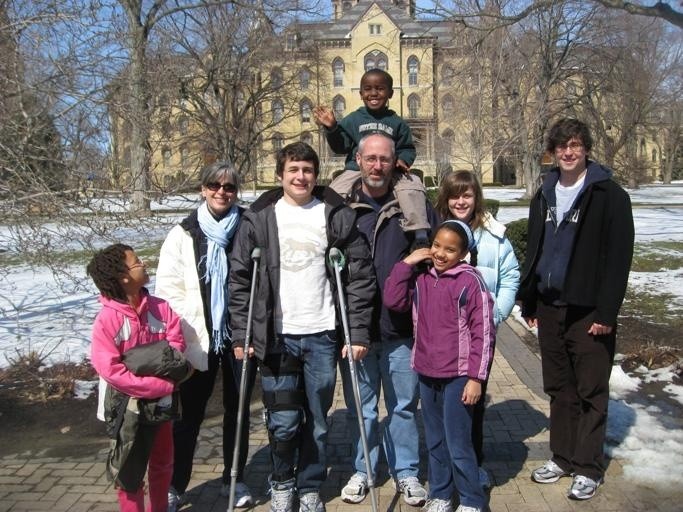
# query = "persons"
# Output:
<box><xmin>433</xmin><ymin>169</ymin><xmax>522</xmax><ymax>493</ymax></box>
<box><xmin>381</xmin><ymin>217</ymin><xmax>498</xmax><ymax>512</ymax></box>
<box><xmin>514</xmin><ymin>116</ymin><xmax>634</xmax><ymax>502</ymax></box>
<box><xmin>313</xmin><ymin>65</ymin><xmax>437</xmax><ymax>266</ymax></box>
<box><xmin>226</xmin><ymin>139</ymin><xmax>379</xmax><ymax>512</ymax></box>
<box><xmin>154</xmin><ymin>159</ymin><xmax>259</xmax><ymax>511</ymax></box>
<box><xmin>326</xmin><ymin>129</ymin><xmax>440</xmax><ymax>506</ymax></box>
<box><xmin>85</xmin><ymin>243</ymin><xmax>188</xmax><ymax>512</ymax></box>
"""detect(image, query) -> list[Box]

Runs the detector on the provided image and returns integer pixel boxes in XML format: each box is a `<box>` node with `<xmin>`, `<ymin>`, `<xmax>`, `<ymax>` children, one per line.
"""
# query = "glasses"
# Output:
<box><xmin>126</xmin><ymin>258</ymin><xmax>144</xmax><ymax>270</ymax></box>
<box><xmin>556</xmin><ymin>143</ymin><xmax>584</xmax><ymax>153</ymax></box>
<box><xmin>205</xmin><ymin>182</ymin><xmax>237</xmax><ymax>192</ymax></box>
<box><xmin>363</xmin><ymin>156</ymin><xmax>394</xmax><ymax>166</ymax></box>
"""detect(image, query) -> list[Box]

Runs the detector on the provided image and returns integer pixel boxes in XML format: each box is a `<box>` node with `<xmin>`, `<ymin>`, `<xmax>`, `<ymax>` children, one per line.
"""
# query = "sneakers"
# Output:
<box><xmin>168</xmin><ymin>492</ymin><xmax>187</xmax><ymax>512</ymax></box>
<box><xmin>299</xmin><ymin>492</ymin><xmax>326</xmax><ymax>512</ymax></box>
<box><xmin>270</xmin><ymin>488</ymin><xmax>297</xmax><ymax>512</ymax></box>
<box><xmin>454</xmin><ymin>504</ymin><xmax>483</xmax><ymax>512</ymax></box>
<box><xmin>421</xmin><ymin>498</ymin><xmax>457</xmax><ymax>512</ymax></box>
<box><xmin>393</xmin><ymin>476</ymin><xmax>429</xmax><ymax>506</ymax></box>
<box><xmin>531</xmin><ymin>459</ymin><xmax>574</xmax><ymax>483</ymax></box>
<box><xmin>341</xmin><ymin>472</ymin><xmax>375</xmax><ymax>503</ymax></box>
<box><xmin>569</xmin><ymin>474</ymin><xmax>604</xmax><ymax>500</ymax></box>
<box><xmin>221</xmin><ymin>482</ymin><xmax>253</xmax><ymax>507</ymax></box>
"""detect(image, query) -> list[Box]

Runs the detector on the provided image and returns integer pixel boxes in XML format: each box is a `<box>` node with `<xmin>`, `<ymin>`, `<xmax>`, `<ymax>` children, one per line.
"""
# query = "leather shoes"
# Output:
<box><xmin>479</xmin><ymin>465</ymin><xmax>492</xmax><ymax>488</ymax></box>
